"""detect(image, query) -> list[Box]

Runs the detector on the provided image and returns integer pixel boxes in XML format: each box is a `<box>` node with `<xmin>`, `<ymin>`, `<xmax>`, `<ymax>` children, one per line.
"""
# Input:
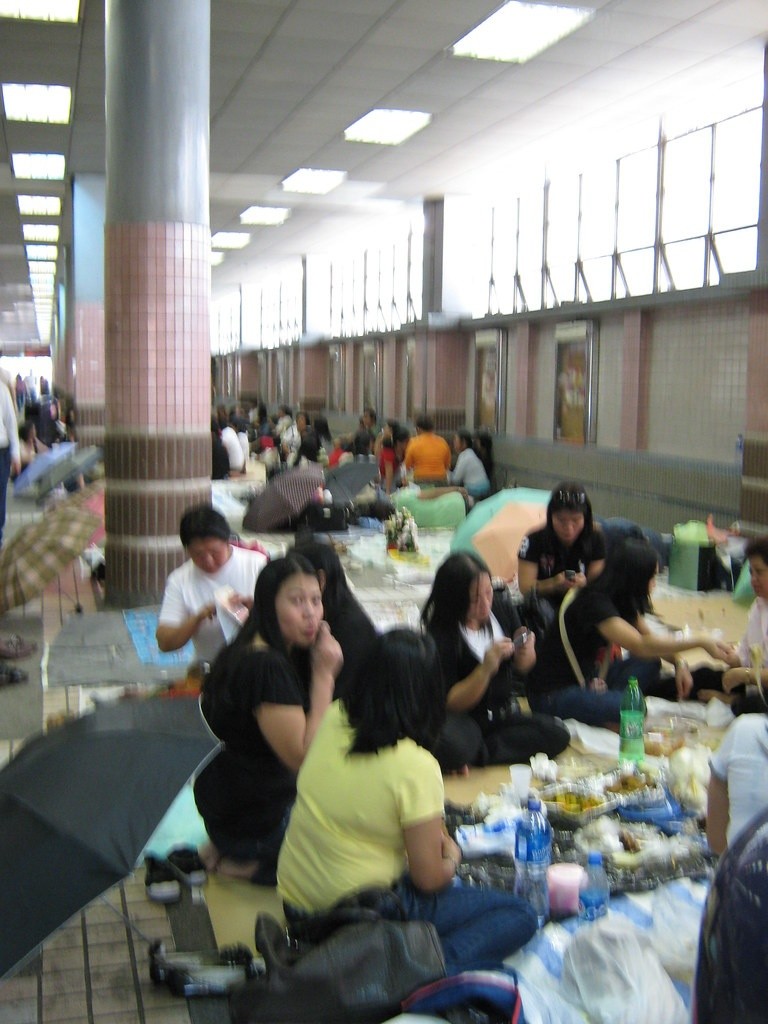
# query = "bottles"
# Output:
<box><xmin>736</xmin><ymin>433</ymin><xmax>744</xmax><ymax>465</ymax></box>
<box><xmin>515</xmin><ymin>799</ymin><xmax>551</xmax><ymax>928</ymax></box>
<box><xmin>385</xmin><ymin>506</ymin><xmax>418</xmax><ymax>558</ymax></box>
<box><xmin>577</xmin><ymin>852</ymin><xmax>609</xmax><ymax>932</ymax></box>
<box><xmin>619</xmin><ymin>676</ymin><xmax>648</xmax><ymax>762</ymax></box>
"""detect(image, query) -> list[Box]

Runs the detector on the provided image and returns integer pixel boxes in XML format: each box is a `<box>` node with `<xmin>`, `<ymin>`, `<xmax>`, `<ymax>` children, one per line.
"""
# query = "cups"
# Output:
<box><xmin>548</xmin><ymin>862</ymin><xmax>584</xmax><ymax>923</ymax></box>
<box><xmin>509</xmin><ymin>762</ymin><xmax>531</xmax><ymax>795</ymax></box>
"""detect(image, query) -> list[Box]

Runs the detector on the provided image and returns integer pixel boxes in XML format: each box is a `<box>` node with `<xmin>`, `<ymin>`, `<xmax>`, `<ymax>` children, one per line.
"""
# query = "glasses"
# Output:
<box><xmin>554</xmin><ymin>489</ymin><xmax>587</xmax><ymax>505</ymax></box>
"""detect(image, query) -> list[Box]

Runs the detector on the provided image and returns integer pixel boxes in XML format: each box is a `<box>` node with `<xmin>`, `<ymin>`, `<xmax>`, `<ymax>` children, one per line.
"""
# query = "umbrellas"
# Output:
<box><xmin>241</xmin><ymin>466</ymin><xmax>323</xmax><ymax>533</ymax></box>
<box><xmin>450</xmin><ymin>485</ymin><xmax>553</xmax><ymax>585</ymax></box>
<box><xmin>0</xmin><ymin>443</ymin><xmax>105</xmax><ymax>613</ymax></box>
<box><xmin>0</xmin><ymin>696</ymin><xmax>223</xmax><ymax>980</ymax></box>
<box><xmin>326</xmin><ymin>463</ymin><xmax>378</xmax><ymax>508</ymax></box>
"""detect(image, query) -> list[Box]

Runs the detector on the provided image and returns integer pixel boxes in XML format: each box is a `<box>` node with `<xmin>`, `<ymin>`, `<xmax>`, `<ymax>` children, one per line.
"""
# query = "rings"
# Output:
<box><xmin>724</xmin><ymin>684</ymin><xmax>727</xmax><ymax>687</ymax></box>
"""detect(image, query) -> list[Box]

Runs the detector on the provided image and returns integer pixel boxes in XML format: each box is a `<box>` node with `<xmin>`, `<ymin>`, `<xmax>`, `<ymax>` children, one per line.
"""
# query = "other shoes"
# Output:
<box><xmin>167</xmin><ymin>846</ymin><xmax>207</xmax><ymax>884</ymax></box>
<box><xmin>145</xmin><ymin>856</ymin><xmax>181</xmax><ymax>903</ymax></box>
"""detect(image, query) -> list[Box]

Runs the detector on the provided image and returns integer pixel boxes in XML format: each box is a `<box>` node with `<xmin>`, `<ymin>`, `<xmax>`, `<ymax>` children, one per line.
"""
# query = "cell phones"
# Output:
<box><xmin>565</xmin><ymin>571</ymin><xmax>575</xmax><ymax>583</ymax></box>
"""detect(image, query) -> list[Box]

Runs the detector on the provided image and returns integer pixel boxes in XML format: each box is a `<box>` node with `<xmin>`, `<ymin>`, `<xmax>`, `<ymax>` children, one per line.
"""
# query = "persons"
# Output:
<box><xmin>0</xmin><ymin>368</ymin><xmax>66</xmax><ymax>545</ymax></box>
<box><xmin>157</xmin><ymin>507</ymin><xmax>268</xmax><ymax>665</ymax></box>
<box><xmin>720</xmin><ymin>537</ymin><xmax>768</xmax><ymax>715</ymax></box>
<box><xmin>706</xmin><ymin>709</ymin><xmax>768</xmax><ymax>860</ymax></box>
<box><xmin>525</xmin><ymin>537</ymin><xmax>734</xmax><ymax>726</ymax></box>
<box><xmin>419</xmin><ymin>552</ymin><xmax>570</xmax><ymax>775</ymax></box>
<box><xmin>276</xmin><ymin>627</ymin><xmax>538</xmax><ymax>978</ymax></box>
<box><xmin>192</xmin><ymin>541</ymin><xmax>379</xmax><ymax>886</ymax></box>
<box><xmin>517</xmin><ymin>481</ymin><xmax>607</xmax><ymax>626</ymax></box>
<box><xmin>211</xmin><ymin>398</ymin><xmax>496</xmax><ymax>528</ymax></box>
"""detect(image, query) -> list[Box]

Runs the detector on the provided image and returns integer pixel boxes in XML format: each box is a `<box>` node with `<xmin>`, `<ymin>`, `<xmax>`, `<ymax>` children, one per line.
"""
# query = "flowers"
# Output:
<box><xmin>385</xmin><ymin>508</ymin><xmax>418</xmax><ymax>554</ymax></box>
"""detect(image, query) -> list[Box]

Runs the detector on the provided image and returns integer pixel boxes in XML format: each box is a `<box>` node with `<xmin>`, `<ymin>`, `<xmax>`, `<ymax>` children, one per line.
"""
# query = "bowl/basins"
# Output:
<box><xmin>643</xmin><ymin>714</ymin><xmax>708</xmax><ymax>756</ymax></box>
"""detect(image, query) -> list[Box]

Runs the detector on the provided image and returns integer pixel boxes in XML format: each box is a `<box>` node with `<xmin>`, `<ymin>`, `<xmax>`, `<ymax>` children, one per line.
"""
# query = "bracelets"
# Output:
<box><xmin>446</xmin><ymin>856</ymin><xmax>457</xmax><ymax>873</ymax></box>
<box><xmin>746</xmin><ymin>668</ymin><xmax>749</xmax><ymax>682</ymax></box>
<box><xmin>675</xmin><ymin>659</ymin><xmax>688</xmax><ymax>668</ymax></box>
